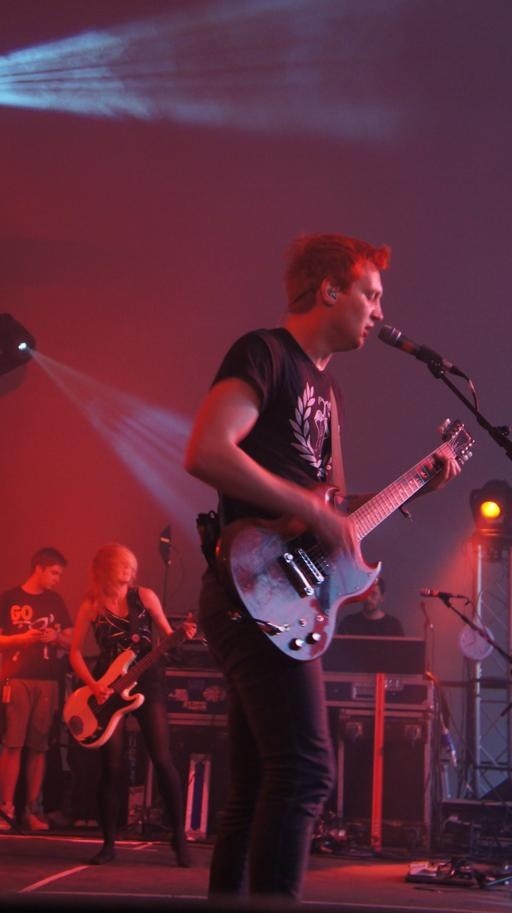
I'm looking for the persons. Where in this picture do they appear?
[0,546,76,832]
[184,226,463,912]
[70,543,197,870]
[338,577,406,672]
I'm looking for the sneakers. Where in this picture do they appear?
[0,804,14,830]
[20,812,48,832]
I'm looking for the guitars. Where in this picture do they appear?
[63,608,203,750]
[215,418,476,661]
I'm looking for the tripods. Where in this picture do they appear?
[122,578,181,843]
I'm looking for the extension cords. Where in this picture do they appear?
[406,871,475,886]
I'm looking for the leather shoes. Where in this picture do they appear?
[170,833,191,866]
[89,845,116,863]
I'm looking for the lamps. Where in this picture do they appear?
[0,315,36,375]
[469,480,512,538]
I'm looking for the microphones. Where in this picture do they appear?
[420,588,464,599]
[378,324,465,378]
[420,601,433,629]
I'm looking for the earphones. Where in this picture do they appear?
[328,288,336,299]
